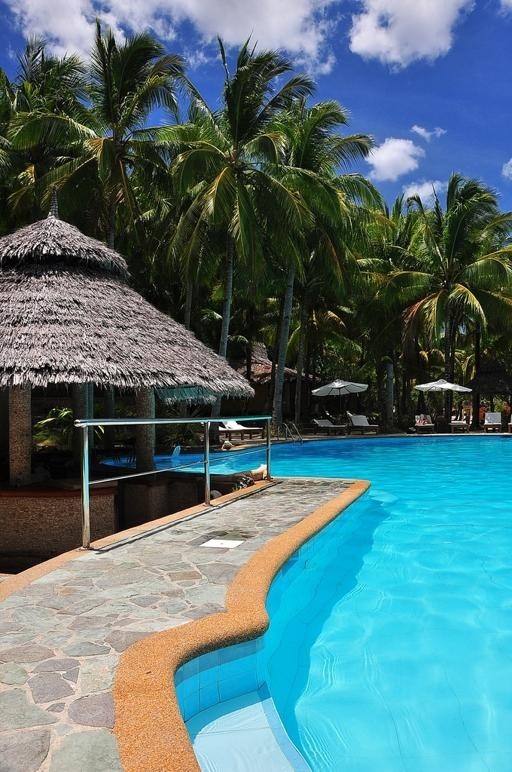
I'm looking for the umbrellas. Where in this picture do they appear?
[413,379,472,417]
[311,378,368,424]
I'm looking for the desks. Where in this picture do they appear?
[415,423,435,434]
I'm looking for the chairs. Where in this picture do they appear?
[313,418,347,436]
[218,426,245,441]
[484,412,502,433]
[222,421,265,439]
[415,414,433,434]
[507,414,512,434]
[114,473,201,533]
[347,414,379,435]
[171,446,181,457]
